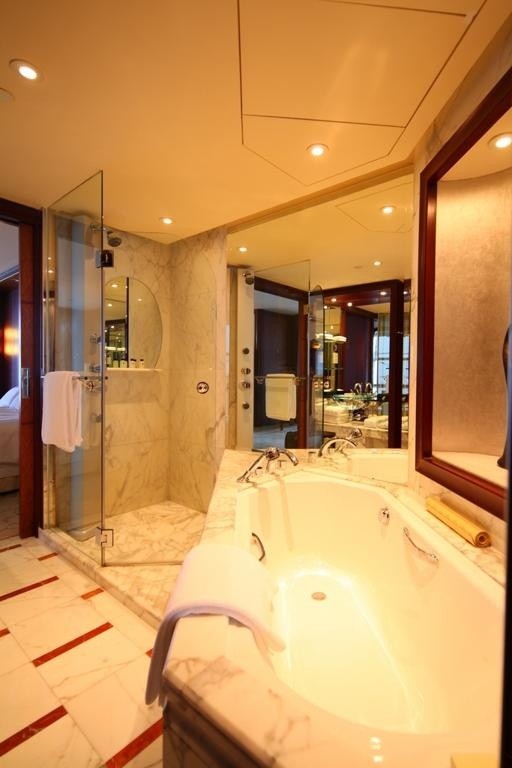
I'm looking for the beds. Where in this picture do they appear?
[0,385,20,492]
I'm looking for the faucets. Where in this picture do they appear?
[317,427,362,464]
[236,445,299,484]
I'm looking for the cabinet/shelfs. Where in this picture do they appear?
[158,683,257,767]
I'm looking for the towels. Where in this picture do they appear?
[40,369,84,452]
[264,371,298,421]
[144,538,290,706]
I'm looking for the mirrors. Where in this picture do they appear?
[323,295,410,404]
[101,274,164,369]
[224,174,419,452]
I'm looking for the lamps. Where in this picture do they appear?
[317,300,347,346]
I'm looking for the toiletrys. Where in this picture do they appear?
[106,350,145,368]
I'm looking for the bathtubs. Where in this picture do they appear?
[158,449,505,768]
